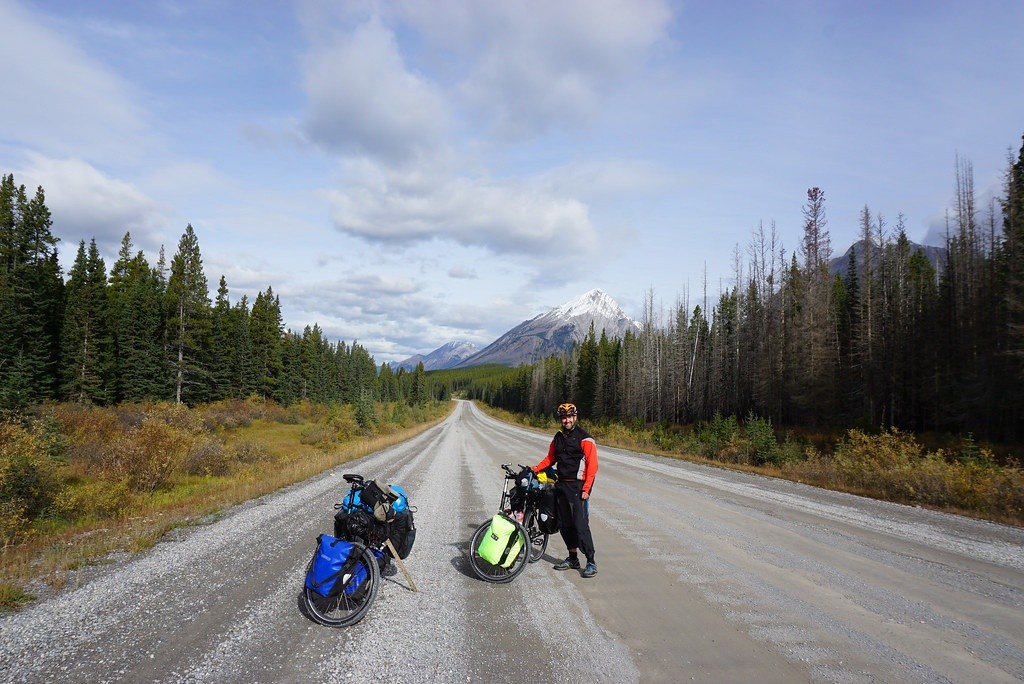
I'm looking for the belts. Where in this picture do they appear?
[559,479,576,481]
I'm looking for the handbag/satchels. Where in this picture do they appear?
[510,466,559,512]
[305,534,386,604]
[334,485,418,559]
[478,515,524,570]
[538,484,560,534]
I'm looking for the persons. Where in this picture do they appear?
[529,403,598,577]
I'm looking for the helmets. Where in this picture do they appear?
[557,403,577,417]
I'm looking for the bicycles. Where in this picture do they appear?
[467,463,550,583]
[299,473,400,629]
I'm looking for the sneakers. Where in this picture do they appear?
[554,557,580,570]
[582,562,598,577]
